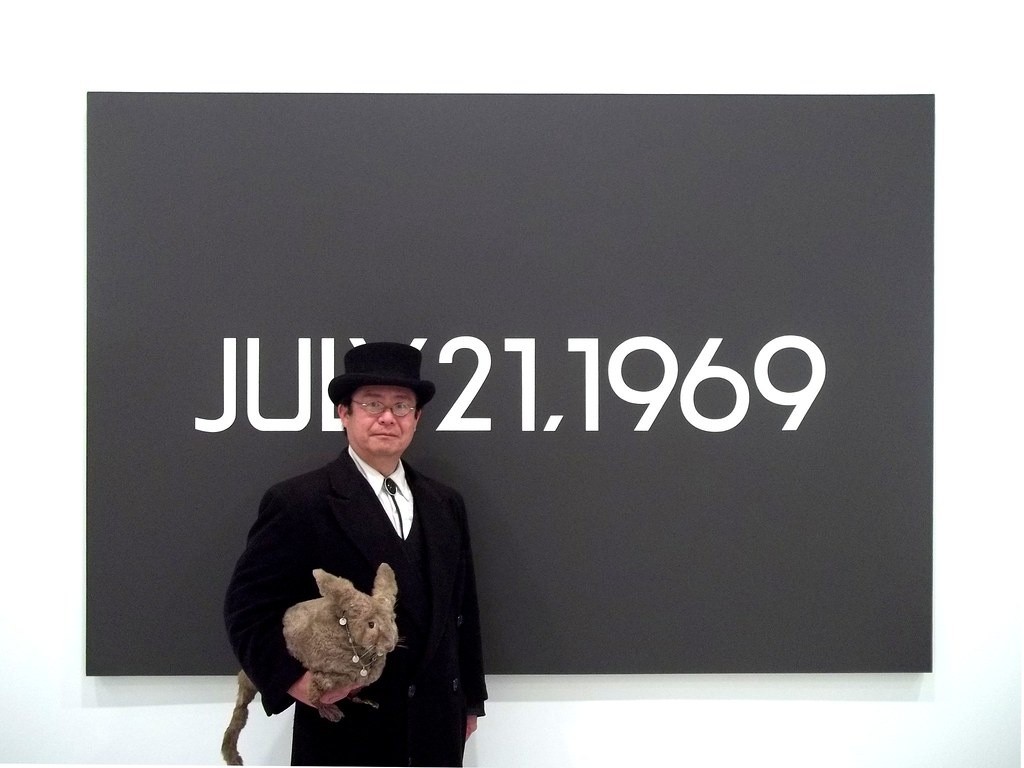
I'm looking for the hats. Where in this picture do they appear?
[328,342,435,411]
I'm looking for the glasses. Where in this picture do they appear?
[352,400,416,417]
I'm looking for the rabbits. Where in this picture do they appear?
[218,562,410,766]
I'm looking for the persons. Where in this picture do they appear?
[224,341,487,766]
